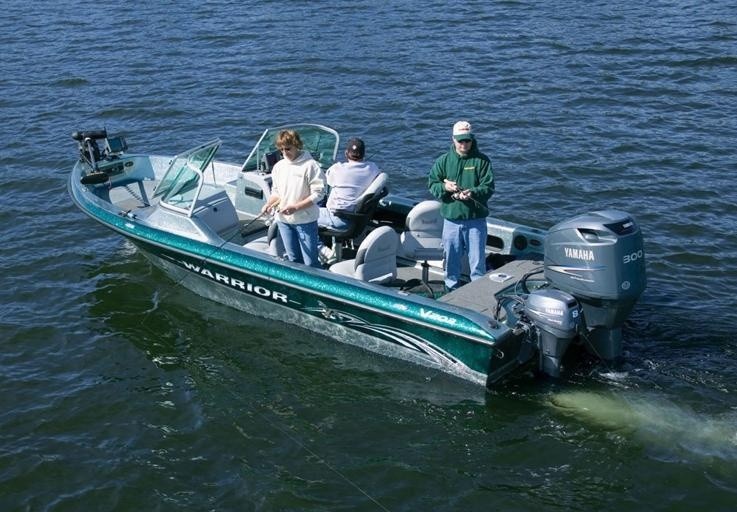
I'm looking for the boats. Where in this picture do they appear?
[62,126,654,402]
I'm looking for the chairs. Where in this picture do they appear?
[330,225,399,286]
[399,200,446,297]
[317,169,388,262]
[244,231,323,263]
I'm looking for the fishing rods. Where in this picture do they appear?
[443,179,504,223]
[363,127,410,162]
[141,199,280,324]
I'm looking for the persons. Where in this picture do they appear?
[316,138,381,266]
[261,129,328,268]
[428,121,495,292]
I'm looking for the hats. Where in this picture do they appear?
[346,138,365,156]
[452,121,473,141]
